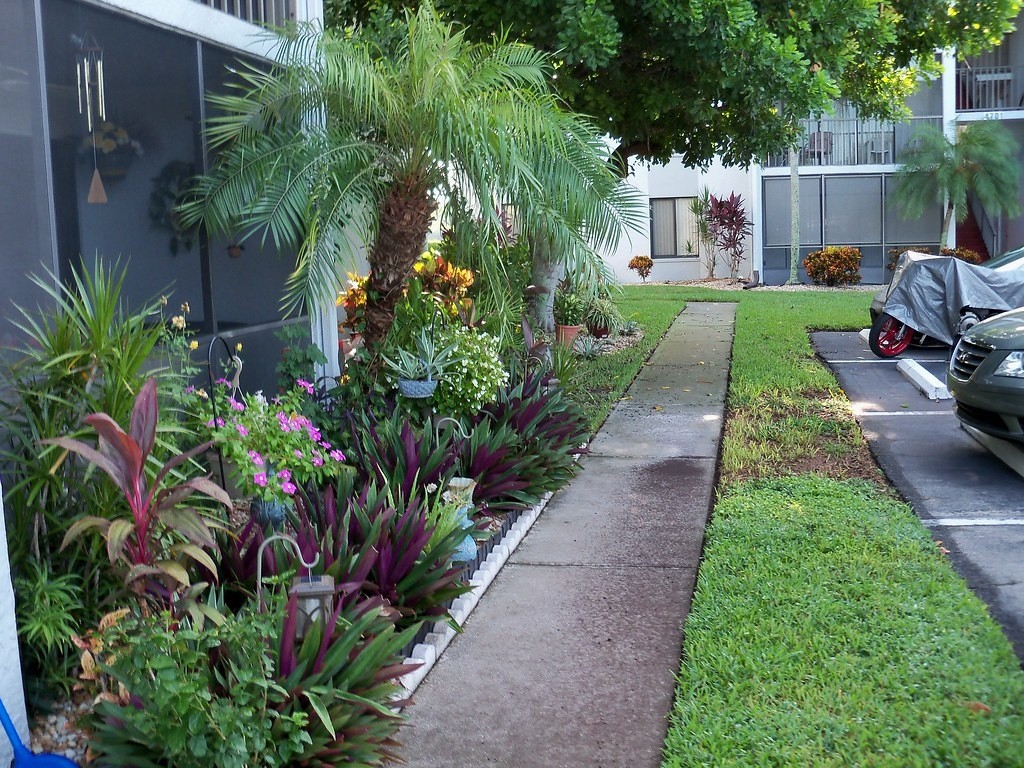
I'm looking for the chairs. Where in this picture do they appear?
[909,139,927,157]
[866,138,890,164]
[801,132,833,165]
[775,148,801,166]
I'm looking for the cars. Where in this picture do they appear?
[945,306,1024,445]
[869,243,1024,348]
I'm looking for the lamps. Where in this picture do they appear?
[289,576,334,641]
[543,378,562,396]
[447,476,478,561]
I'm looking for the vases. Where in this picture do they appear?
[206,451,267,499]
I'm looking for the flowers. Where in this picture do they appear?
[160,296,347,499]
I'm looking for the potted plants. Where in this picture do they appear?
[554,292,586,349]
[380,325,473,399]
[585,299,623,339]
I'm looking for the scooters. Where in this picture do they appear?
[868,250,957,359]
